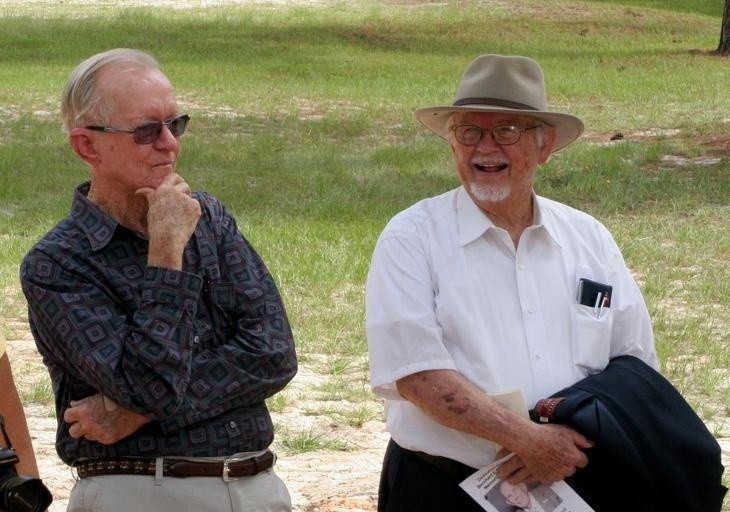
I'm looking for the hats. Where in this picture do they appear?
[414,54,583,153]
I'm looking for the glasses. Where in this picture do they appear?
[84,114,190,144]
[448,123,542,146]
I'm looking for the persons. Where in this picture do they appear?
[365,53,660,511]
[20,54,298,512]
[499,478,560,510]
[0,329,52,512]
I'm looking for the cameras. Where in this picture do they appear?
[0,447,53,512]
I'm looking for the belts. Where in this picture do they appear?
[76,450,278,482]
